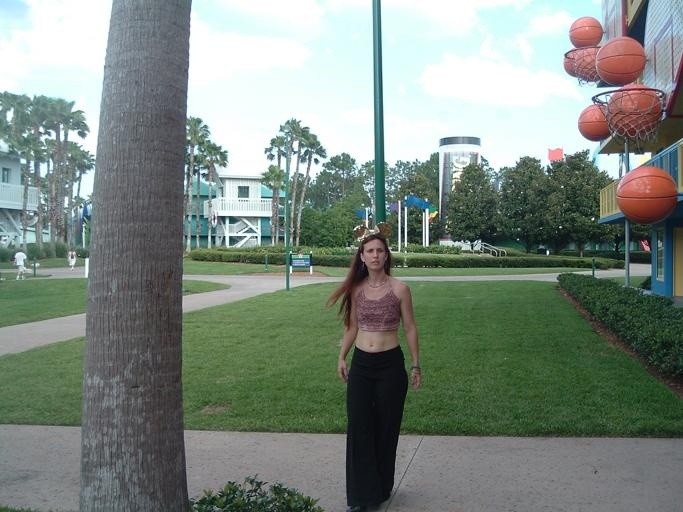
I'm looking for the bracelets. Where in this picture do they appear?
[410,367,420,375]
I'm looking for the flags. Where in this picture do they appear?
[82,203,89,228]
[428,204,437,222]
[355,209,365,219]
[548,148,563,161]
[405,195,429,208]
[389,203,398,213]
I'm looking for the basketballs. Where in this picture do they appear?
[615,167,678,224]
[564,18,661,141]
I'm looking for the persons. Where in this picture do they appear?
[323,232,421,512]
[13,248,27,280]
[66,246,77,271]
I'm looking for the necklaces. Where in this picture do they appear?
[365,275,387,289]
